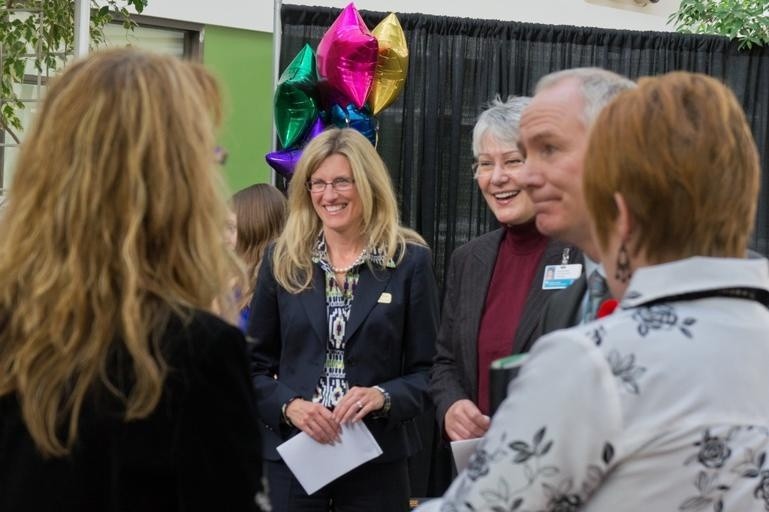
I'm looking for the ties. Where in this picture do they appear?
[584,271,608,320]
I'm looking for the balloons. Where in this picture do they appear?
[265,1,410,182]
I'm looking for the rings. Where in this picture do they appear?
[356,400,363,409]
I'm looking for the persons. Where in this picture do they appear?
[241,126,439,512]
[214,184,289,333]
[432,94,583,442]
[0,45,264,510]
[411,69,767,512]
[515,68,636,346]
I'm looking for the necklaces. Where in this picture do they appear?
[332,251,367,273]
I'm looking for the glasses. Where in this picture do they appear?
[471,158,527,176]
[306,176,354,193]
